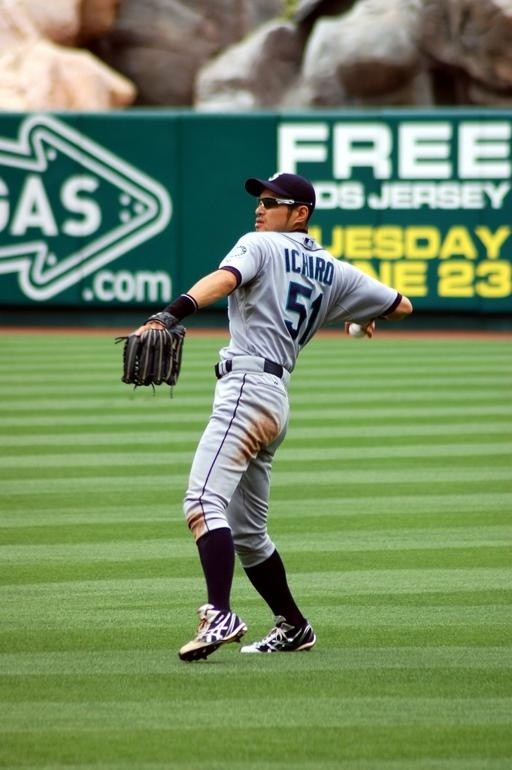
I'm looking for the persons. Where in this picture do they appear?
[124,171,416,661]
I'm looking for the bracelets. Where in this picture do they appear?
[163,291,199,327]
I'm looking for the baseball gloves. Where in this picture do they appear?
[114,312,185,398]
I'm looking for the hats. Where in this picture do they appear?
[244,171,317,211]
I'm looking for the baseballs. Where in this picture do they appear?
[349,323,366,337]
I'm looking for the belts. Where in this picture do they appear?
[213,355,292,387]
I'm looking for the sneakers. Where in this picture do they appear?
[178,603,248,662]
[240,615,317,654]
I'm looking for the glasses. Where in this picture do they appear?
[258,197,313,210]
